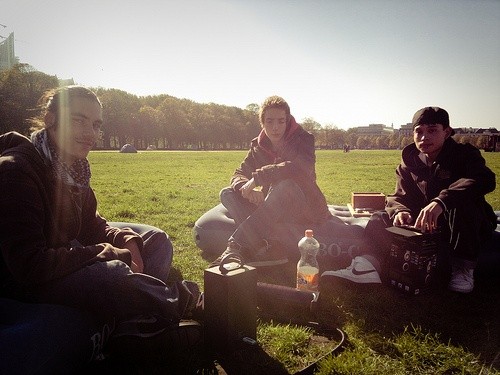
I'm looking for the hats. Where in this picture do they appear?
[413,108,456,135]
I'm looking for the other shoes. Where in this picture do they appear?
[106,316,204,361]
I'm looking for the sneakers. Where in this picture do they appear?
[319,255,381,291]
[449,264,476,292]
[212,234,250,265]
[244,237,288,267]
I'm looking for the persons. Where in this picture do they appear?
[0,86,202,337]
[319,107,498,294]
[208,98,328,269]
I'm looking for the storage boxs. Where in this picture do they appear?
[351,192,386,212]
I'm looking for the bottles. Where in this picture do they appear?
[296,230,320,302]
